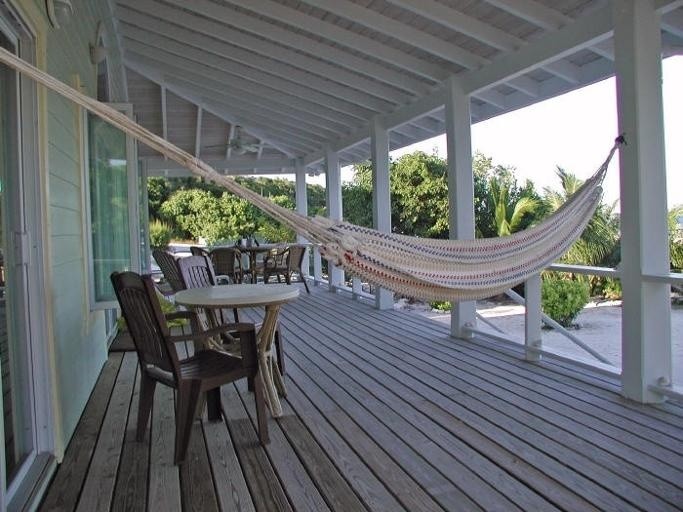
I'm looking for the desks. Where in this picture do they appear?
[175,282,300,419]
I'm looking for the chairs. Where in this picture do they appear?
[190,236,312,294]
[104,249,286,466]
